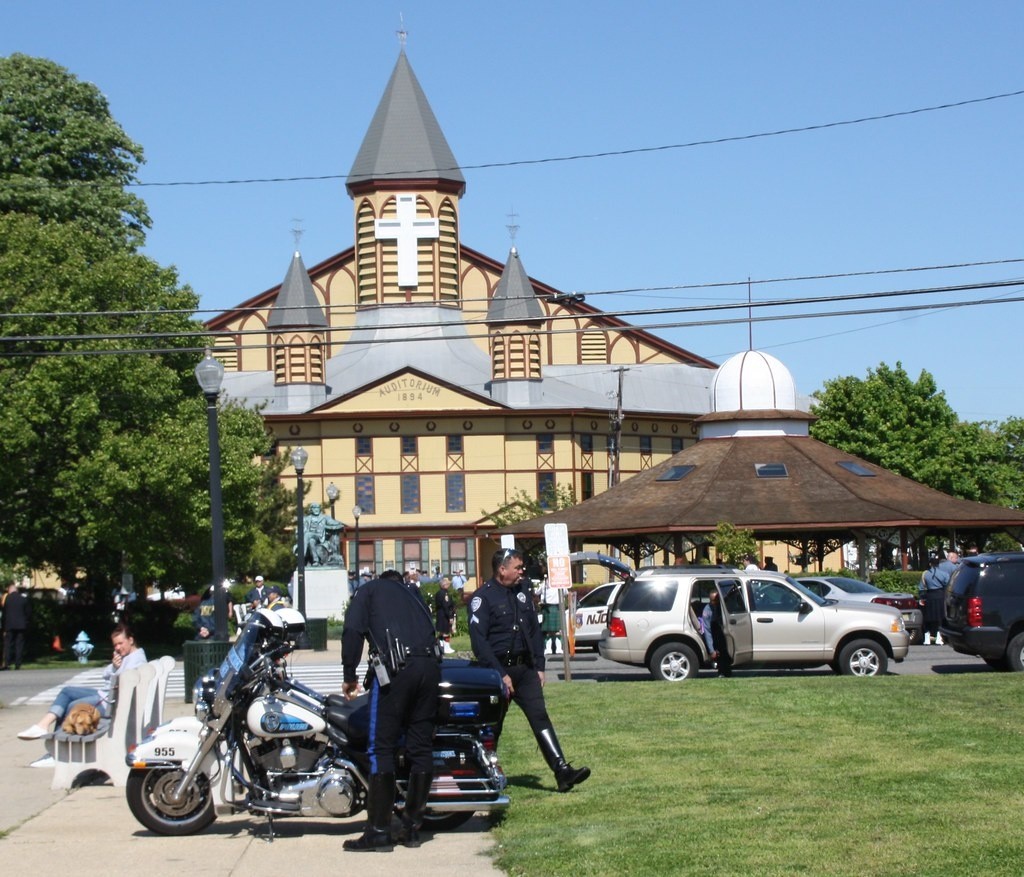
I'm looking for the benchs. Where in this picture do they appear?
[234,601,253,636]
[38,653,176,790]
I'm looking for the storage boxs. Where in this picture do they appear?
[439,659,505,725]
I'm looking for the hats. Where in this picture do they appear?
[265,585,280,597]
[255,575,263,581]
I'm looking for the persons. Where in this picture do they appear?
[17,626,148,768]
[466,548,591,793]
[347,567,442,593]
[245,576,269,608]
[702,590,732,677]
[763,556,777,571]
[743,555,760,570]
[919,555,949,645]
[193,587,233,641]
[342,569,439,851]
[940,546,978,574]
[114,599,125,623]
[533,578,568,653]
[435,578,456,654]
[0,586,32,671]
[267,585,284,611]
[453,570,466,597]
[303,504,345,566]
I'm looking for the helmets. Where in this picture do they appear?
[257,609,284,637]
[276,607,306,632]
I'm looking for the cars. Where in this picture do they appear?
[939,552,1024,672]
[566,550,923,681]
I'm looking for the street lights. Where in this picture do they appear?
[195,344,230,643]
[327,481,338,520]
[352,504,361,582]
[291,445,308,618]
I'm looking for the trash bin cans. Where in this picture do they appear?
[183,641,235,704]
[539,603,560,632]
[306,619,328,650]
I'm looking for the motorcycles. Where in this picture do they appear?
[125,608,511,841]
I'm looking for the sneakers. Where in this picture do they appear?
[17,725,49,740]
[30,753,55,767]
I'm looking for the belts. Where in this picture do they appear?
[383,646,435,662]
[499,654,533,666]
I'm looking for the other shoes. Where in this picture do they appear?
[718,669,727,678]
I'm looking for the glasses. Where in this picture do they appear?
[502,548,513,564]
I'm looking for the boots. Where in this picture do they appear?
[533,726,591,791]
[936,631,944,644]
[442,640,455,653]
[342,773,394,852]
[440,640,444,647]
[923,631,930,645]
[394,771,434,847]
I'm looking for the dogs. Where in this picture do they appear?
[61,702,101,735]
